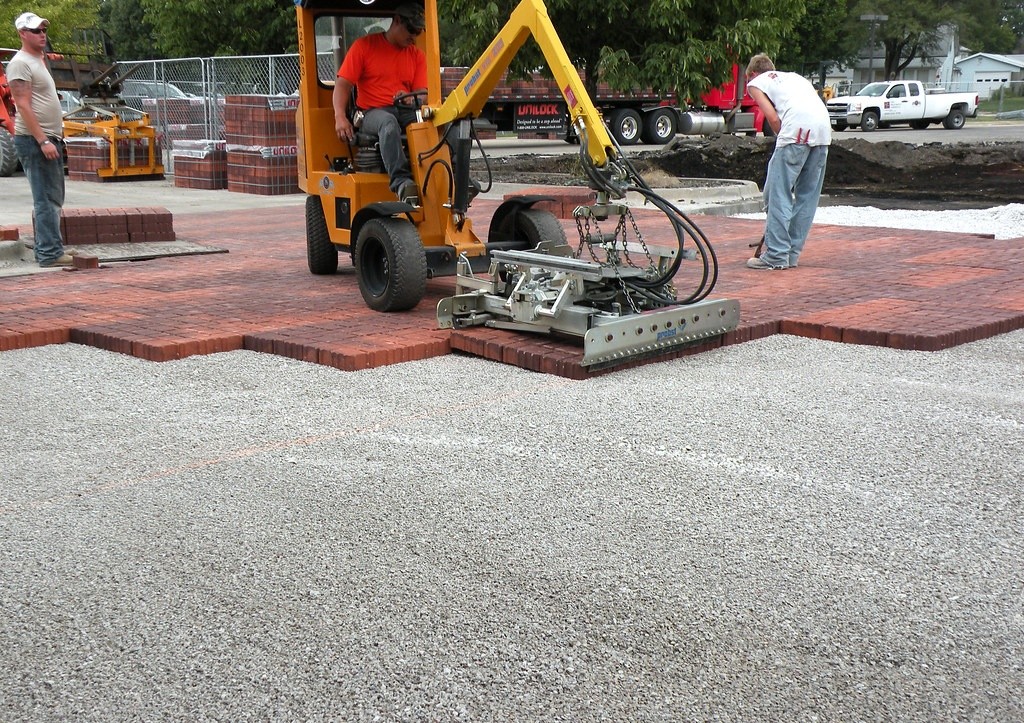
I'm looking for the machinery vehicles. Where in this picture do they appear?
[0,27,164,179]
[295,1,741,366]
[813,82,836,104]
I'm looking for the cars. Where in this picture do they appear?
[123,82,202,108]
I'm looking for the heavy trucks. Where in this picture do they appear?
[440,42,778,145]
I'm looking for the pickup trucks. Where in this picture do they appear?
[826,80,979,132]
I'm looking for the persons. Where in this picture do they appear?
[5,11,77,266]
[332,3,482,207]
[745,54,832,268]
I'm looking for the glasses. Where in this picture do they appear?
[23,28,47,34]
[399,14,421,36]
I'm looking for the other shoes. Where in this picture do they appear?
[747,257,786,270]
[40,253,73,266]
[64,249,78,255]
[397,180,415,202]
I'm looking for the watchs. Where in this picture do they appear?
[39,138,51,146]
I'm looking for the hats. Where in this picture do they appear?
[14,13,50,29]
[398,2,425,29]
